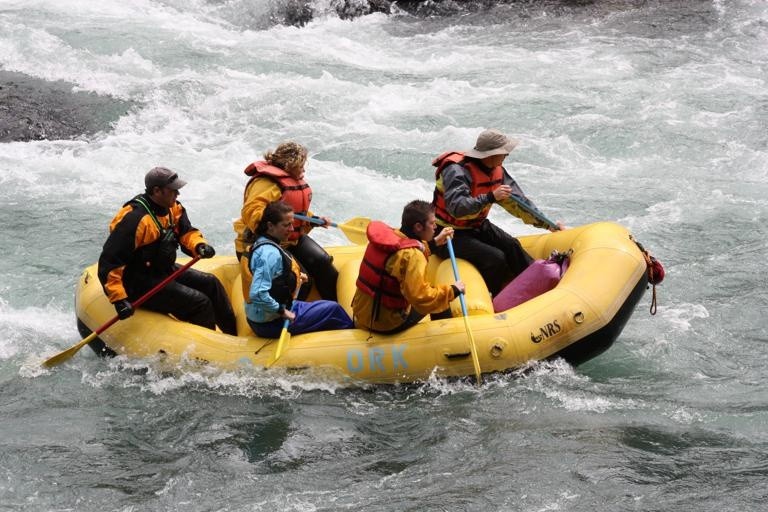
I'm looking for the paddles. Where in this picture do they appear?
[445,234,482,387]
[293,214,371,246]
[261,276,302,368]
[38,255,201,368]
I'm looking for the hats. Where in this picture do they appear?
[145,166,186,192]
[464,130,518,160]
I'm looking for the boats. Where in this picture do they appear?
[74,217,669,382]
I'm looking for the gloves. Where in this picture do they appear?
[197,243,214,258]
[115,300,134,320]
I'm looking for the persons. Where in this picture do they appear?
[98,166,237,336]
[349,199,465,335]
[240,200,355,338]
[233,140,337,302]
[426,130,565,299]
